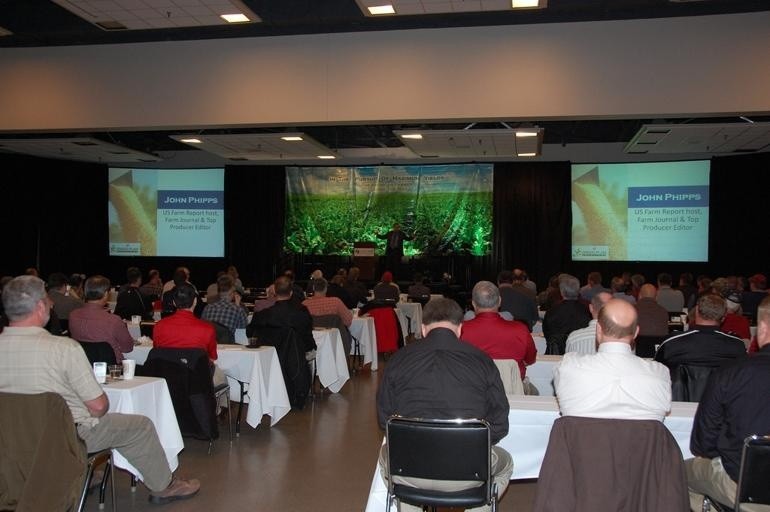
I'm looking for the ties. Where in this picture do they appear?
[391,231,397,249]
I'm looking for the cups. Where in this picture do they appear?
[352,308,360,317]
[680,315,688,325]
[121,360,136,378]
[132,315,141,324]
[249,337,257,344]
[93,362,107,383]
[108,365,123,380]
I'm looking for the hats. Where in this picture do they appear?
[750,273,767,289]
[383,271,393,282]
[497,270,513,281]
[710,278,729,292]
[724,289,745,303]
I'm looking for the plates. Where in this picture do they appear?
[245,345,261,348]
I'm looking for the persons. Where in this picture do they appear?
[697,276,745,292]
[552,298,673,420]
[583,271,611,300]
[656,273,684,312]
[497,270,538,332]
[542,275,593,337]
[142,268,163,295]
[523,270,537,293]
[26,267,39,278]
[654,293,747,361]
[247,275,317,351]
[114,267,151,319]
[284,269,305,301]
[460,280,539,395]
[46,273,83,320]
[71,273,85,300]
[408,272,430,297]
[328,274,353,309]
[375,297,514,512]
[0,275,201,505]
[565,291,637,354]
[632,283,670,336]
[508,267,536,305]
[348,267,368,297]
[743,274,767,310]
[201,274,248,343]
[375,223,416,256]
[611,271,645,303]
[206,271,227,304]
[163,267,200,295]
[686,295,751,339]
[70,274,134,364]
[374,271,398,299]
[683,297,770,512]
[163,271,201,316]
[152,285,218,361]
[337,268,348,274]
[228,266,243,288]
[301,277,353,327]
[306,269,323,293]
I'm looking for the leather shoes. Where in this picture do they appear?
[88,470,105,495]
[148,476,201,505]
